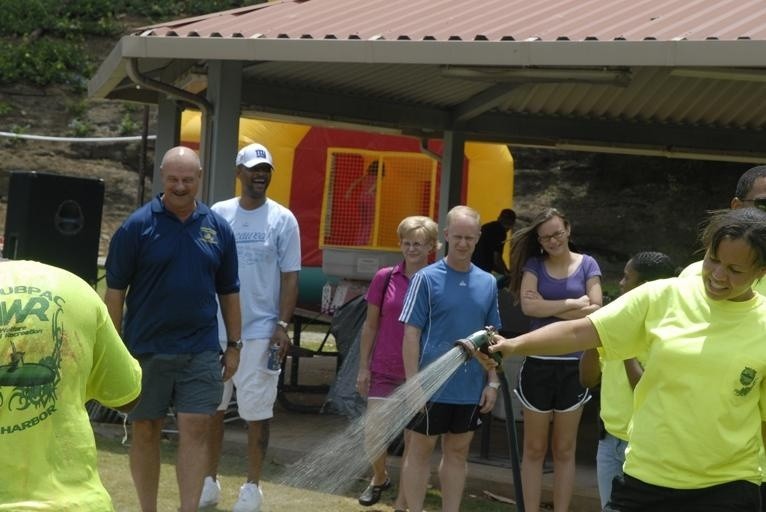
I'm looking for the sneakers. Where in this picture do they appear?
[198,475,223,509]
[231,482,264,511]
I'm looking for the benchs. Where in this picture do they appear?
[277,344,318,412]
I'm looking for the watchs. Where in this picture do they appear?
[487,382,502,392]
[226,340,243,350]
[277,320,289,332]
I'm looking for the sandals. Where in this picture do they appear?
[358,475,392,506]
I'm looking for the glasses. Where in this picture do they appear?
[536,227,565,243]
[739,196,766,211]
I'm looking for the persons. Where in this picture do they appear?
[579,252,684,509]
[0,258,144,512]
[346,161,385,247]
[198,144,301,512]
[105,145,242,512]
[355,215,442,505]
[397,204,503,512]
[677,165,765,299]
[503,207,604,512]
[471,209,517,291]
[474,207,765,512]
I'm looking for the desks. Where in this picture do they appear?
[292,308,335,386]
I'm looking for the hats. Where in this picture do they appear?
[236,142,275,173]
